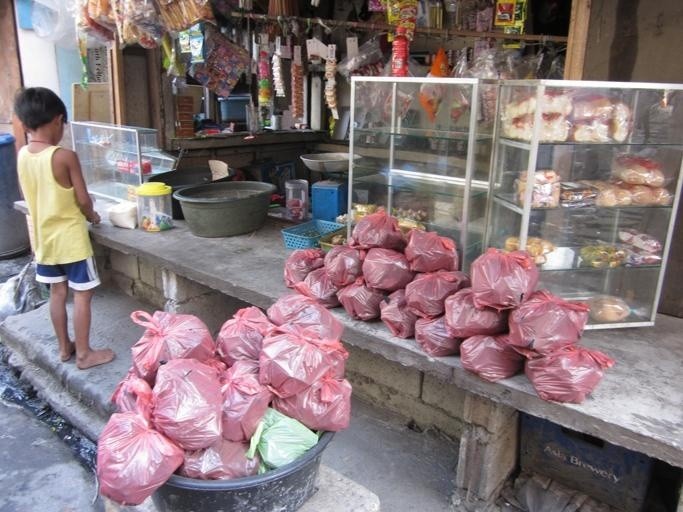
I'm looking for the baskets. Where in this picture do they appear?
[281,218,345,249]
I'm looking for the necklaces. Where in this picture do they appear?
[30,139,51,145]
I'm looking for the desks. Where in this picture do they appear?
[10,195,683,512]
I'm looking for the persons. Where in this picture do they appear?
[12,84,116,369]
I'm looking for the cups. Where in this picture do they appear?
[284,179,309,222]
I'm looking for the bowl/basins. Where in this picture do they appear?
[170,180,278,239]
[298,152,361,173]
[146,427,336,512]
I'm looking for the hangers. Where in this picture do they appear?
[222,10,567,68]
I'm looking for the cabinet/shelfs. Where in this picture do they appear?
[484,77,681,329]
[67,119,172,208]
[350,77,499,271]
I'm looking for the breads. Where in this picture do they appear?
[505,237,554,265]
[502,92,674,209]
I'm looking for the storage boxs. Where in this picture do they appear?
[217,97,249,121]
